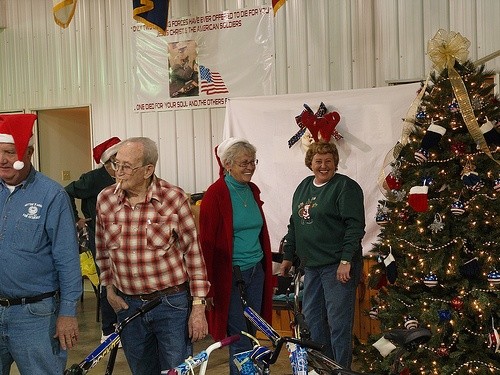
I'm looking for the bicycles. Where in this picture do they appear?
[232,264,325,375]
[62,295,163,375]
[286,300,431,375]
[160,333,242,375]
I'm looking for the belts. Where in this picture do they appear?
[0,287,60,308]
[128,280,190,302]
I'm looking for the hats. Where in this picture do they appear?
[0,114,38,170]
[214,137,249,178]
[93,137,122,164]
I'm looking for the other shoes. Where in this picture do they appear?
[100,331,122,347]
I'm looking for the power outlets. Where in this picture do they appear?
[62,170,70,181]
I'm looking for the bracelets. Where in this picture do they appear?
[341,260,350,264]
[193,300,207,306]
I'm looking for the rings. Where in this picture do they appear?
[203,334,206,335]
[71,335,75,339]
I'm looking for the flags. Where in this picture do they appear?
[53,0,77,29]
[272,0,286,17]
[133,0,170,36]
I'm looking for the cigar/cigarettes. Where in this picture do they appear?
[114,179,122,194]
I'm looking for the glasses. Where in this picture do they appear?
[233,159,258,167]
[110,162,152,173]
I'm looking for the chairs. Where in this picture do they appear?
[79,223,101,323]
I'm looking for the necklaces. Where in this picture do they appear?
[225,176,249,207]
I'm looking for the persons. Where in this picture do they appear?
[65,137,123,348]
[280,142,366,375]
[95,137,210,375]
[198,140,273,375]
[0,114,82,375]
